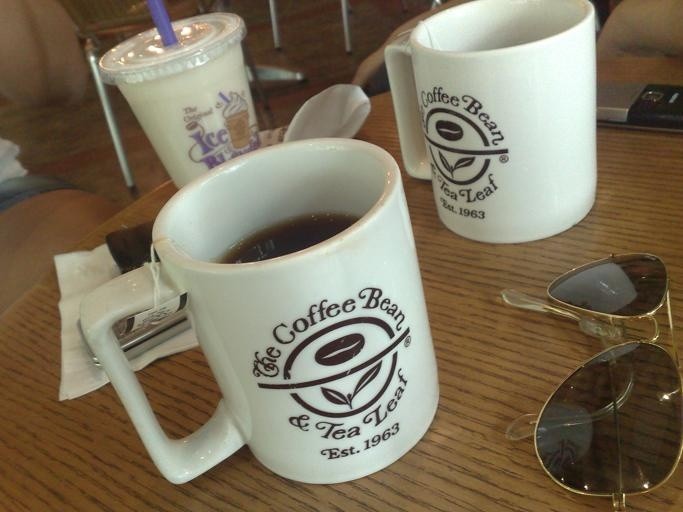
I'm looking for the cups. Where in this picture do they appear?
[95,12,265,199]
[74,133,442,487]
[382,0,598,246]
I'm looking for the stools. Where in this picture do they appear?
[0,189,118,314]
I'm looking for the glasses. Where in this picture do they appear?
[491,251,683,512]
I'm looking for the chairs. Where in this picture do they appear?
[53,0,273,195]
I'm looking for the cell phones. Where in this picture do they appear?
[76,292,191,369]
[597,82,683,129]
